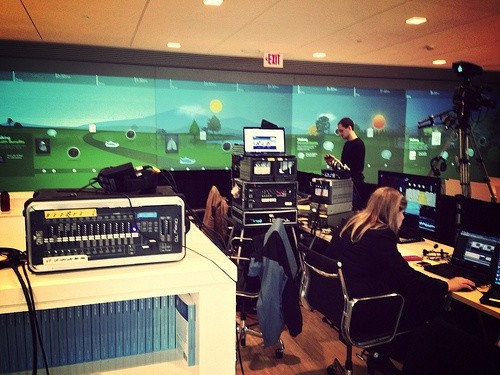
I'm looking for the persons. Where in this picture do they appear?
[324,118,365,210]
[327,186,475,375]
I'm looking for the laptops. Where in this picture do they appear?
[243,126,297,159]
[480,252,500,309]
[424,225,500,287]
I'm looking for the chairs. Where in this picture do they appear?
[296,242,435,375]
[229,218,285,359]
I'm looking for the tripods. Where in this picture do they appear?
[417,84,499,203]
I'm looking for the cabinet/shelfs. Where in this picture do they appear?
[0,216,240,375]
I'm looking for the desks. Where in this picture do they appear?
[296,213,500,320]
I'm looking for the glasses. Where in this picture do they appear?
[338,128,345,133]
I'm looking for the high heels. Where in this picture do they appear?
[366,356,383,375]
[383,360,411,375]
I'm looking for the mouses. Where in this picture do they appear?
[454,284,476,292]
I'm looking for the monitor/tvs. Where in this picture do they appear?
[377,169,441,244]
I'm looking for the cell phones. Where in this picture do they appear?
[415,261,430,269]
